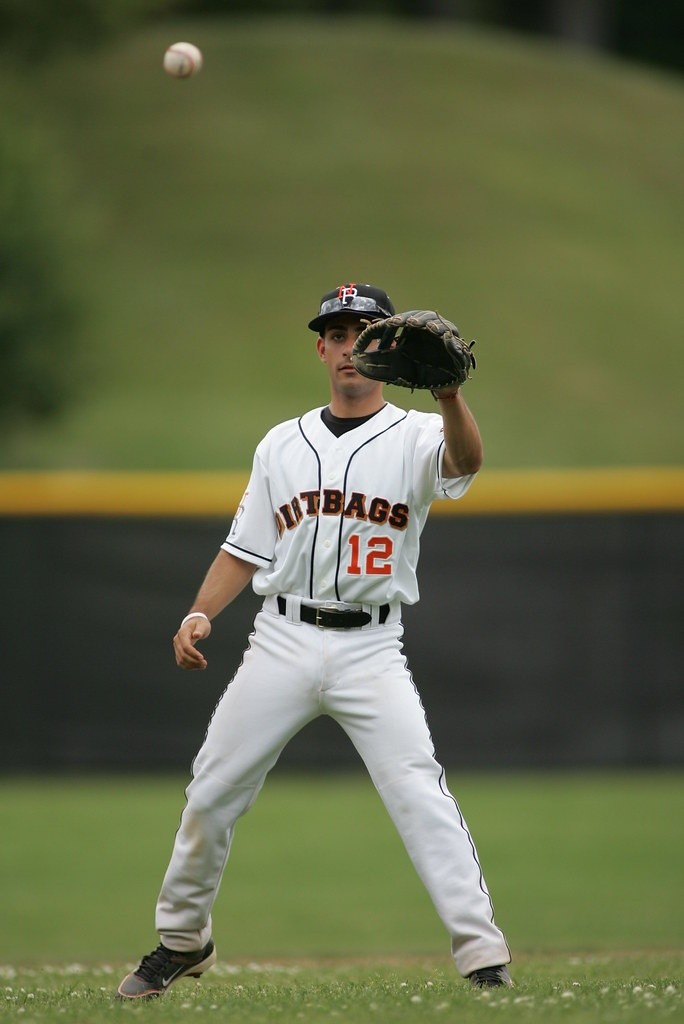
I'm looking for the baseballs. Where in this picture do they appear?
[162,42,204,80]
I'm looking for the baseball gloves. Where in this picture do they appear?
[353,308,477,396]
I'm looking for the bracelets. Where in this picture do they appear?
[181,611,209,628]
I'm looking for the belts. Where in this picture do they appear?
[277,595,390,628]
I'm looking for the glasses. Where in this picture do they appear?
[317,296,392,316]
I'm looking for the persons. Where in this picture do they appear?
[116,285,516,1002]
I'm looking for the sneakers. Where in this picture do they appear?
[469,965,511,989]
[116,937,216,1002]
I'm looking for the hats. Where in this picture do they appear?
[308,284,395,332]
